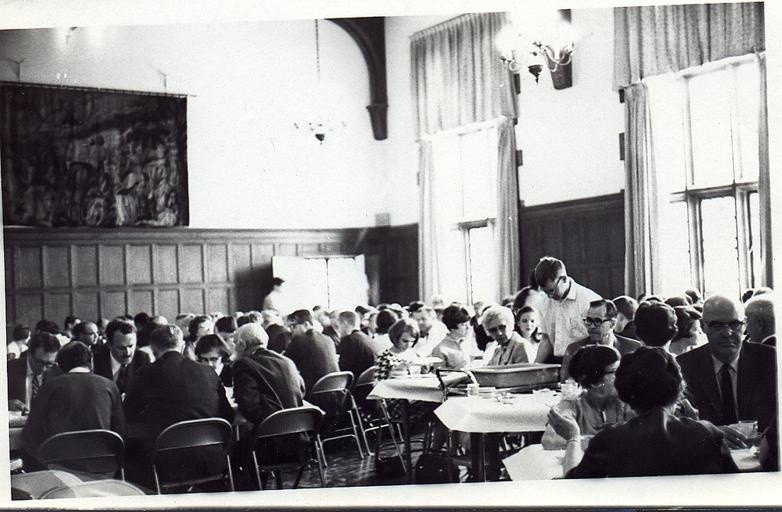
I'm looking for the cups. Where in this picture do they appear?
[466,383,480,396]
[739,420,758,440]
[409,365,421,379]
[560,384,574,391]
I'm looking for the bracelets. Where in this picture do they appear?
[566,439,581,446]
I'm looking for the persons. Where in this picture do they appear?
[534,257,604,363]
[542,344,636,449]
[615,288,779,471]
[263,277,286,312]
[547,346,738,479]
[559,299,641,387]
[7,306,382,481]
[372,269,540,466]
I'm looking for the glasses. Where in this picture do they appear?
[582,316,616,327]
[599,367,619,376]
[488,324,507,333]
[543,275,566,295]
[287,322,300,330]
[199,352,223,363]
[30,354,57,369]
[701,318,745,332]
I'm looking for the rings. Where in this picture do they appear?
[726,433,732,438]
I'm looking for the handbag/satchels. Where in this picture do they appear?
[413,448,461,484]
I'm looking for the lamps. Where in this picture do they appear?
[491,9,583,92]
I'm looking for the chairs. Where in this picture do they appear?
[10,300,782,498]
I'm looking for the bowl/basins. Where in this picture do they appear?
[478,386,496,399]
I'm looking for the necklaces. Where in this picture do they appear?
[588,393,609,423]
